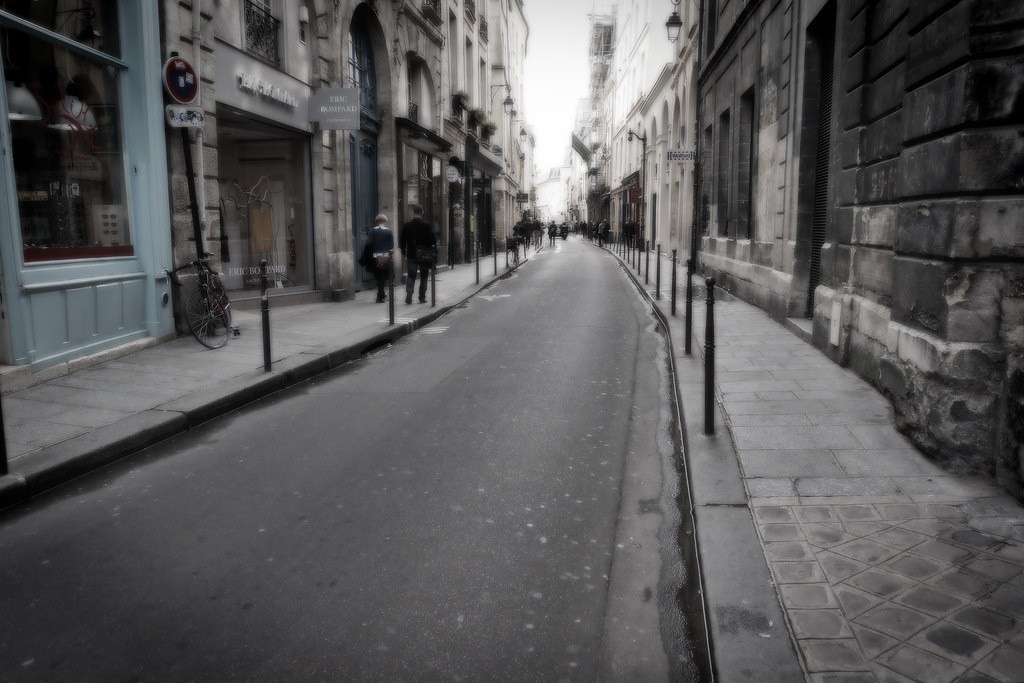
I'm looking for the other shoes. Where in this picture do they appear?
[376,295,387,303]
[405,301,411,304]
[420,300,427,303]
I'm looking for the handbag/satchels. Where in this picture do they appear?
[416,248,437,264]
[377,256,394,270]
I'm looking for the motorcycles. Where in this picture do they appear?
[559,226,568,240]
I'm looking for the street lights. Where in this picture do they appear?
[627,127,647,239]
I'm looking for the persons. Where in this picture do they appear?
[510,211,635,250]
[362,213,396,303]
[400,204,438,304]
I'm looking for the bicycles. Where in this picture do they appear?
[506,235,520,272]
[165,251,240,349]
